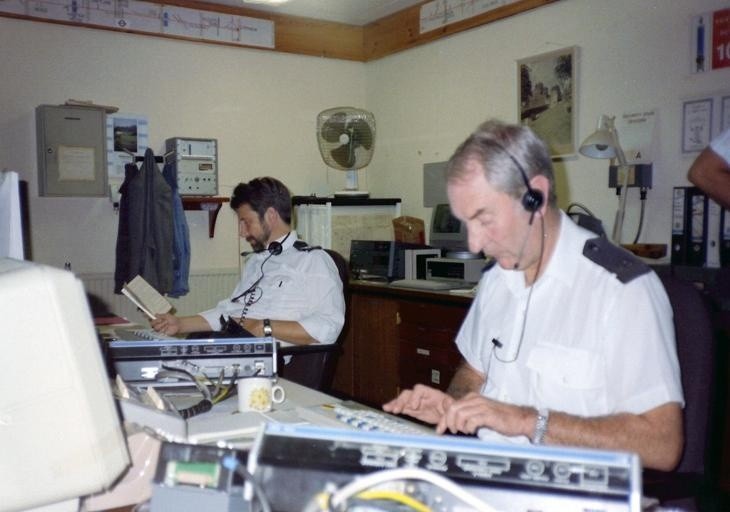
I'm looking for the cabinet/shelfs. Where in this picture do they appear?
[319,283,476,436]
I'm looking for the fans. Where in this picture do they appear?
[317,107,376,199]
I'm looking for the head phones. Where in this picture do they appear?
[268,232,290,255]
[486,138,543,212]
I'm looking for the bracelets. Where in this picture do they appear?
[531,405,551,444]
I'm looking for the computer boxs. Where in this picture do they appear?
[426,257,488,283]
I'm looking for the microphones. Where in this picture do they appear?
[241,247,276,256]
[514,200,540,270]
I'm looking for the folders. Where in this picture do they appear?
[672,187,730,270]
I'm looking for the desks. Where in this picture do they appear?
[80,372,688,511]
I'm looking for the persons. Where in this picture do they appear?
[140,174,347,387]
[685,124,729,214]
[381,118,689,473]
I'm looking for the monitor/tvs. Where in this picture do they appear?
[429,202,476,259]
[0,255,131,511]
[0,170,25,260]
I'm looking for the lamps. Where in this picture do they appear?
[578,113,631,247]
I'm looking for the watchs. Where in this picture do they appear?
[261,318,274,340]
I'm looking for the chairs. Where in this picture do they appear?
[278,248,347,391]
[643,264,716,512]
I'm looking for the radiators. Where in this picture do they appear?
[75,269,240,341]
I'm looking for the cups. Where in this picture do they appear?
[237,376,286,414]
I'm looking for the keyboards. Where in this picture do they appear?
[294,400,442,436]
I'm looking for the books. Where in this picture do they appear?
[120,275,173,322]
